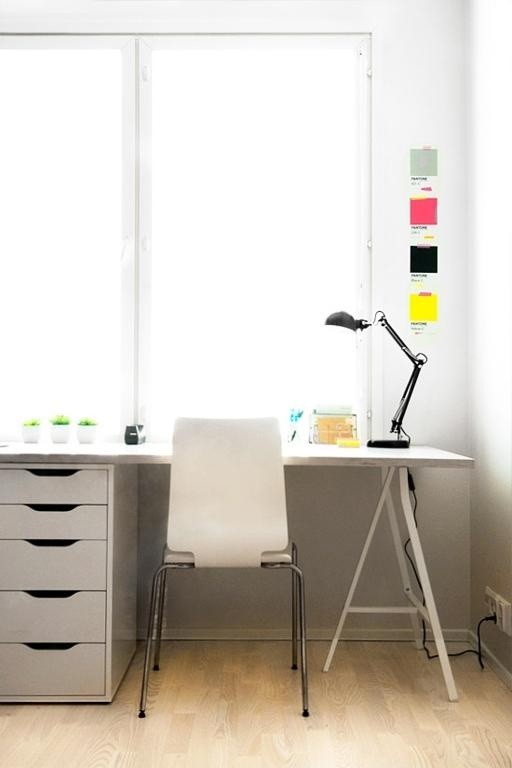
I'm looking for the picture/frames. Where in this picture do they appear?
[301,403,369,452]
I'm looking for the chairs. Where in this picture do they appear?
[136,414,311,720]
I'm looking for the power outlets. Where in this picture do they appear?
[482,584,512,637]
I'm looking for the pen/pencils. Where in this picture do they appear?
[288,409,303,441]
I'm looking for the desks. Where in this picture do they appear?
[1,441,478,705]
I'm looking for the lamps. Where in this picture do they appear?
[319,304,430,452]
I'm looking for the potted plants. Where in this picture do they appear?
[18,414,105,448]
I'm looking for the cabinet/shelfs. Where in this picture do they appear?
[0,462,140,706]
